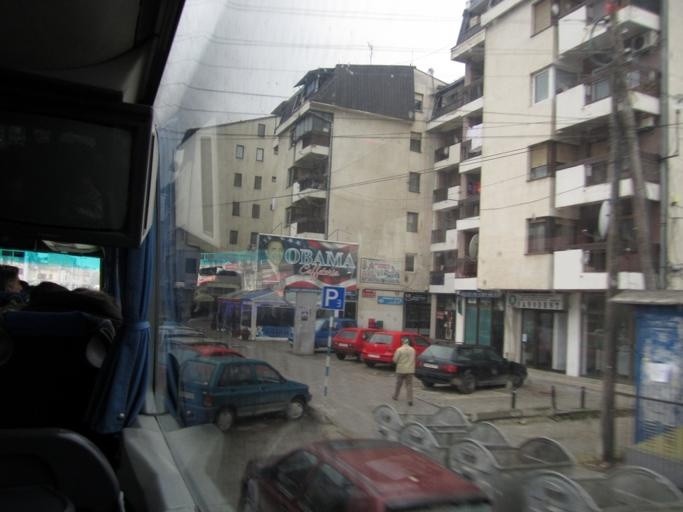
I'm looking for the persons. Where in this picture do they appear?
[390,338,415,406]
[259,239,288,282]
[0,265,26,296]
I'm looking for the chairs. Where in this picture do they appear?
[0,429,124,512]
[0,311,130,453]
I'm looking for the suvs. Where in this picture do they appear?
[287,319,358,352]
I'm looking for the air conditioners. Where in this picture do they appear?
[631,30,658,57]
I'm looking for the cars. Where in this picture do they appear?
[414,342,529,394]
[240,438,490,512]
[175,356,314,431]
[333,327,394,363]
[361,330,436,369]
[155,323,244,388]
